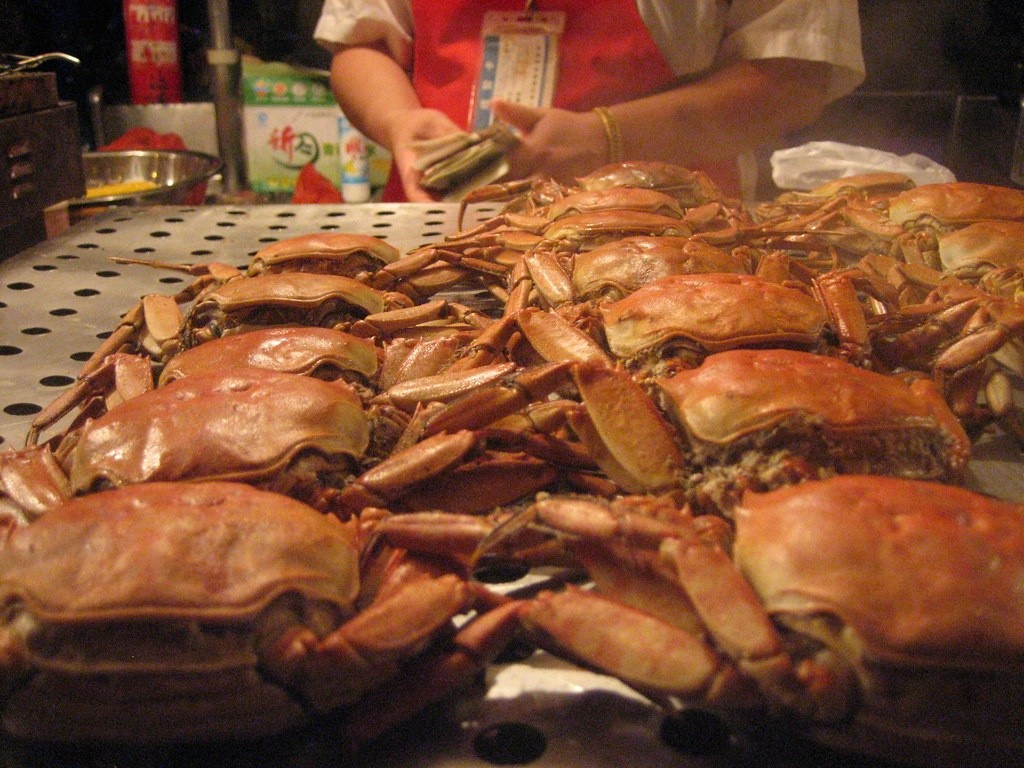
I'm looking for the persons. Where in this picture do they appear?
[313,0,867,203]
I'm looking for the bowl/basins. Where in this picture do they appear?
[67,149,225,226]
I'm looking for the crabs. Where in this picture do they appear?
[1,166,1022,747]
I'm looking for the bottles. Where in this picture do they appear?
[336,103,371,203]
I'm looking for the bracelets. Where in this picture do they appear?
[594,106,621,163]
